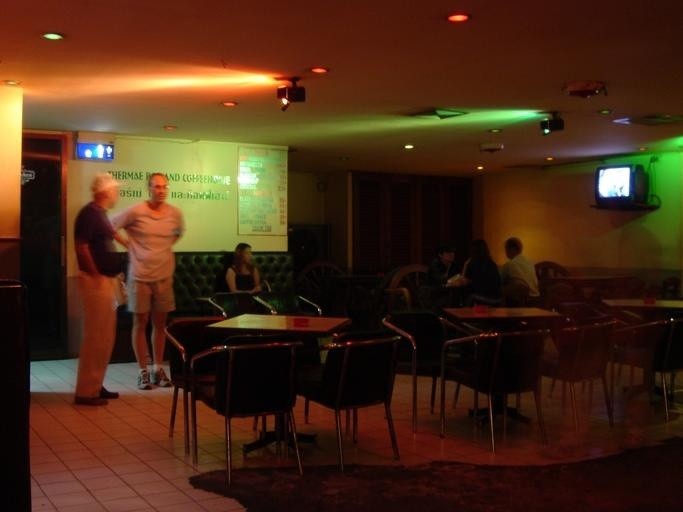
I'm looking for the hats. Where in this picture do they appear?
[91,173,118,196]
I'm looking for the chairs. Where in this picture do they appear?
[163,316,225,456]
[381,310,472,428]
[309,335,403,474]
[190,341,304,487]
[542,324,616,435]
[207,292,323,317]
[609,318,682,422]
[440,330,548,453]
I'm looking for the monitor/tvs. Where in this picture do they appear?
[594,164,647,205]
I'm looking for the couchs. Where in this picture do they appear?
[100,250,317,362]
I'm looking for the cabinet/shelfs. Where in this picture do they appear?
[326,168,481,276]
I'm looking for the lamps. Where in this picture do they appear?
[274,76,307,112]
[538,110,564,136]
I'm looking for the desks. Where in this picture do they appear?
[443,307,562,425]
[206,313,351,455]
[602,299,683,399]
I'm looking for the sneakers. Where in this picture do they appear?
[153,369,172,387]
[138,370,152,390]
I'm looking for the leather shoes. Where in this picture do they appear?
[75,397,107,406]
[100,386,118,398]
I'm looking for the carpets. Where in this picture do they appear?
[191,435,683,512]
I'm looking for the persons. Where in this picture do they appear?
[447,240,501,305]
[73,171,130,406]
[422,241,457,287]
[108,172,185,390]
[501,237,540,299]
[224,243,261,293]
